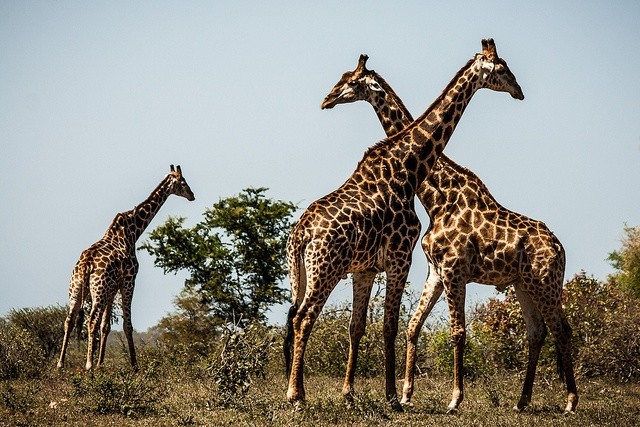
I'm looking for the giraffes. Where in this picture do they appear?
[57,164,195,375]
[321,53,579,415]
[283,38,524,411]
